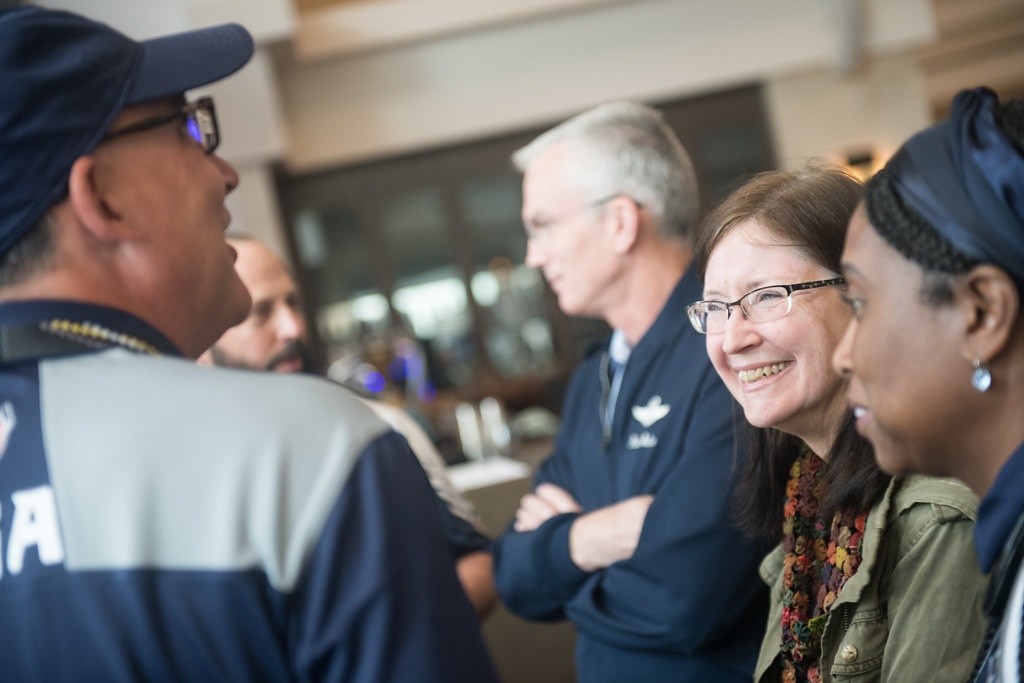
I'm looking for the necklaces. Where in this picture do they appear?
[46,320,160,357]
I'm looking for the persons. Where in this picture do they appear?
[693,162,990,683]
[828,87,1024,683]
[0,6,500,683]
[490,101,803,683]
[184,228,501,636]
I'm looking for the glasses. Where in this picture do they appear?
[101,97,220,154]
[684,277,846,335]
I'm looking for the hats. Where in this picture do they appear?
[878,85,1024,278]
[0,8,254,253]
